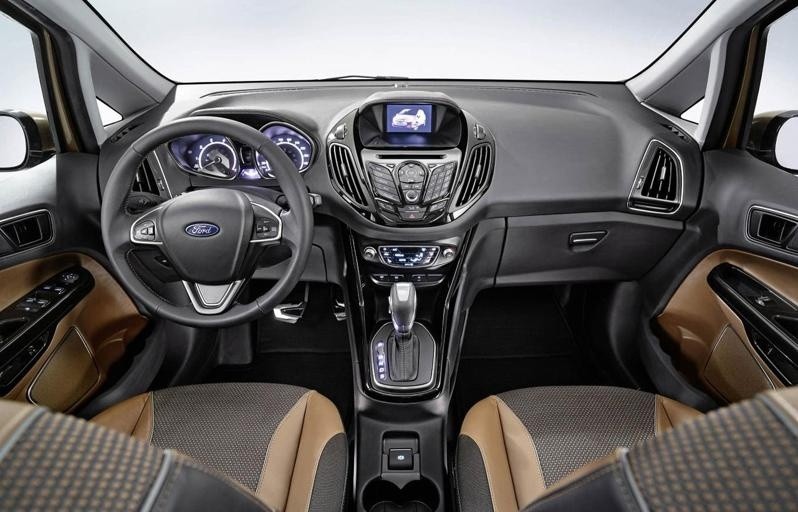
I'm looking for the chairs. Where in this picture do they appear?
[0,383,349,512]
[454,385,798,512]
